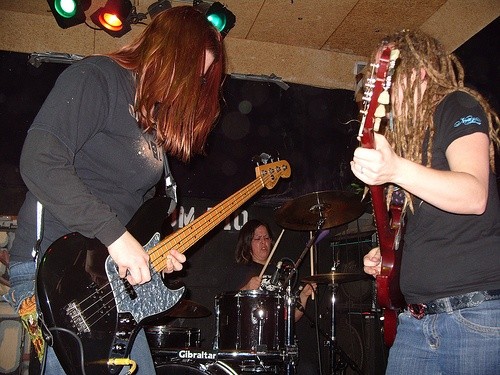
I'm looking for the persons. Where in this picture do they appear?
[217,218,317,322]
[4,5,227,375]
[349,29,500,375]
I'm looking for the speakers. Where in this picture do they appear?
[315,306,388,375]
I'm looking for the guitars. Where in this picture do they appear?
[355,45,407,343]
[35,152,293,374]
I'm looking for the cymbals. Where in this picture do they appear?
[280,188,365,229]
[167,299,213,320]
[299,271,369,284]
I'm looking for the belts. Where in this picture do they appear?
[408,289,500,320]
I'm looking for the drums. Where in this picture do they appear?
[217,291,298,359]
[153,355,239,375]
[142,326,202,350]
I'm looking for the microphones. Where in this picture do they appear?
[270,260,283,285]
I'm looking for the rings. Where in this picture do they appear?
[360,166,364,174]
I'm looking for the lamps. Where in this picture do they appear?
[148,0,172,22]
[89,0,137,40]
[192,0,237,41]
[46,0,92,31]
